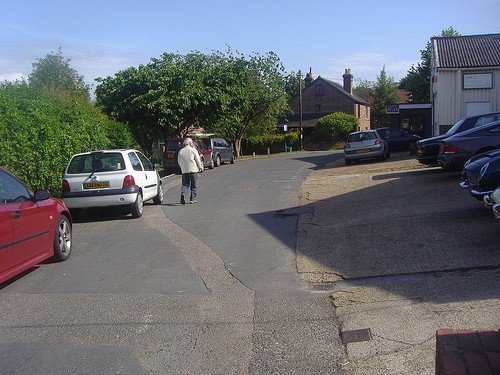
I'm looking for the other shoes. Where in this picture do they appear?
[181,194,186,205]
[190,199,197,204]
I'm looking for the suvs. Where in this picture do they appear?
[164,137,215,171]
[203,136,236,166]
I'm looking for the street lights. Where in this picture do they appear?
[296,69,304,151]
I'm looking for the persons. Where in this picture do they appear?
[178,138,204,205]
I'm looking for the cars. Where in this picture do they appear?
[342,131,388,164]
[484,188,499,218]
[375,127,420,150]
[0,165,75,290]
[64,147,164,216]
[437,121,500,167]
[460,148,500,200]
[414,113,500,166]
